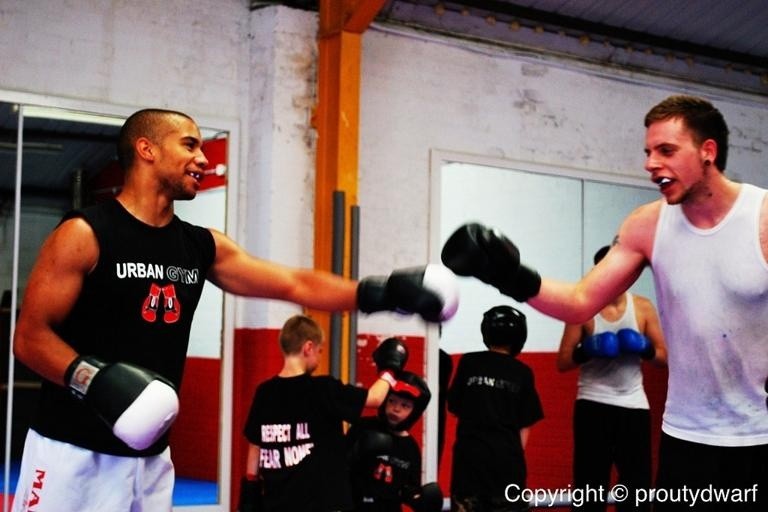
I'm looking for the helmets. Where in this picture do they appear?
[379,370,430,432]
[481,306,527,353]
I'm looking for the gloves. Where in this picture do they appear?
[573,329,655,365]
[373,338,408,389]
[357,263,458,321]
[441,224,541,303]
[64,352,181,450]
[412,482,443,511]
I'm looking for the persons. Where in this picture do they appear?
[344,372,442,512]
[555,245,668,512]
[441,94,768,512]
[439,324,453,464]
[12,109,458,512]
[240,316,408,512]
[448,307,543,512]
